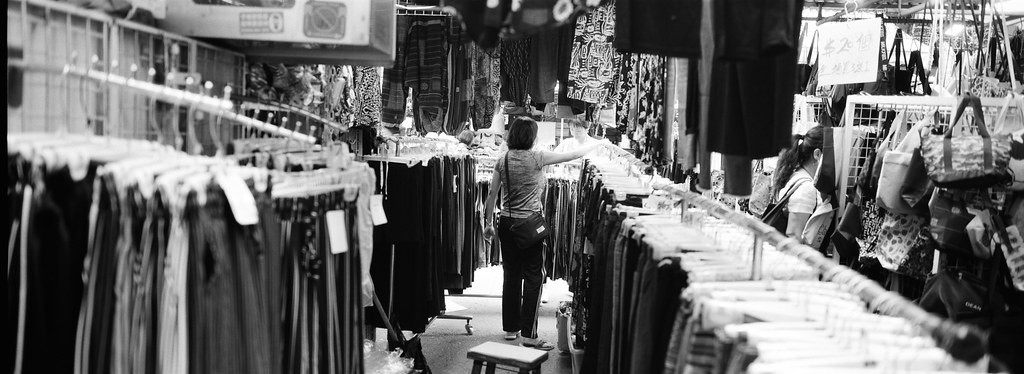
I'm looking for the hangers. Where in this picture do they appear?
[6,56,1007,374]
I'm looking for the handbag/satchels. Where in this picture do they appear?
[798,7,1024,374]
[749,157,771,216]
[510,211,552,251]
[761,176,817,238]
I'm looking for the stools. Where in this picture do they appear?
[466,341,548,374]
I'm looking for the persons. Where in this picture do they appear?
[459,131,475,147]
[771,125,823,242]
[555,119,601,155]
[482,116,611,350]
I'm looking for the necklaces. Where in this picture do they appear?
[801,165,814,179]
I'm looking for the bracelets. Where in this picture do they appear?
[486,219,493,225]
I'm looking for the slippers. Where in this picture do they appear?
[505,330,521,339]
[520,336,553,350]
